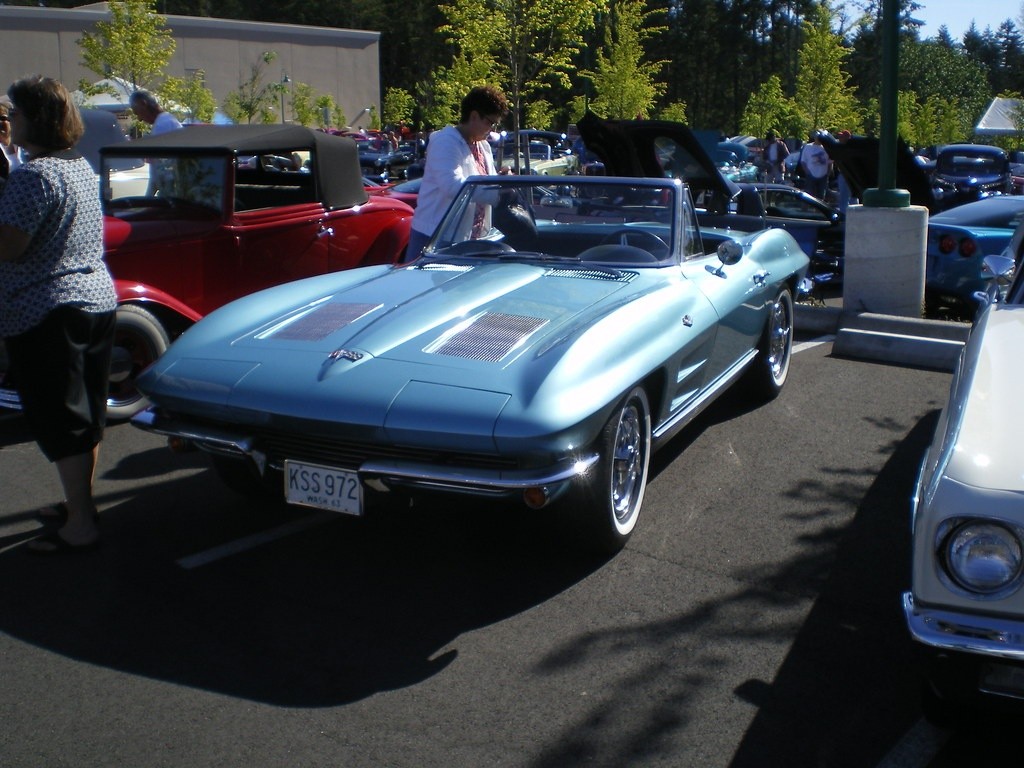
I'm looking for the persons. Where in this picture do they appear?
[763,130,877,213]
[0,75,182,556]
[405,87,515,263]
[358,120,425,151]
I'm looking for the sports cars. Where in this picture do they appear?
[127,175,811,550]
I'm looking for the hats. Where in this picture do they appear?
[837,130,852,139]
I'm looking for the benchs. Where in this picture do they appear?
[526,225,732,259]
[235,184,276,210]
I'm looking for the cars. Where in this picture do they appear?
[898,218,1023,662]
[316,105,1024,317]
[0,123,418,427]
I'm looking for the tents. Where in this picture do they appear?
[69,72,191,114]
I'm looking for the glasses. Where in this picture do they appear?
[479,112,499,129]
[7,108,18,116]
[0,115,8,120]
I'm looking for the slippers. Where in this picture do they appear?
[33,503,99,526]
[27,528,103,557]
